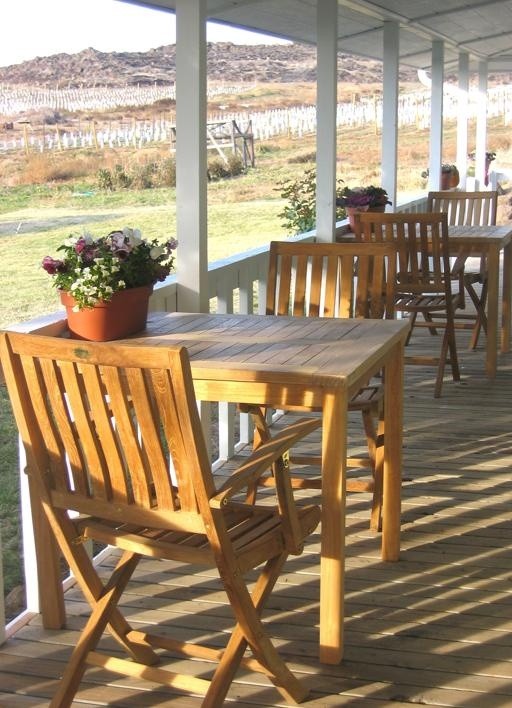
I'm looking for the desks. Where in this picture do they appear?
[24,310,411,665]
[336,225,512,382]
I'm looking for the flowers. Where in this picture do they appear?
[335,185,392,207]
[40,226,177,313]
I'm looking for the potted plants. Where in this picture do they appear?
[468,151,496,187]
[441,164,459,190]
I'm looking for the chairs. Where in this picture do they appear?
[0,328,321,707]
[405,191,497,350]
[234,240,396,534]
[352,212,460,398]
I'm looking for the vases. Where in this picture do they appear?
[53,286,152,341]
